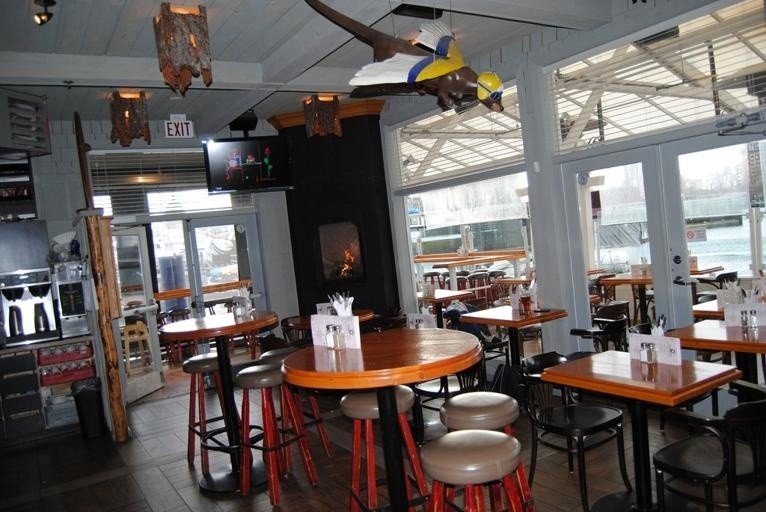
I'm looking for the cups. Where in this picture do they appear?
[37,343,90,377]
[519,295,532,315]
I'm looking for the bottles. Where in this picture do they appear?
[332,325,345,350]
[641,343,648,362]
[233,297,248,316]
[750,309,758,327]
[741,310,749,327]
[647,343,657,363]
[325,324,334,350]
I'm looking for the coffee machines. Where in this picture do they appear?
[0,266,60,347]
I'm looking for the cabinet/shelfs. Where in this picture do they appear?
[1,332,102,454]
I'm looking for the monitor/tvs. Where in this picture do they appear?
[203,133,305,195]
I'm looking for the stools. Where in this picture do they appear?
[121,262,766,511]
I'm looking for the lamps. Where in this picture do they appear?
[106,89,151,148]
[151,1,214,98]
[302,94,343,141]
[31,0,57,26]
